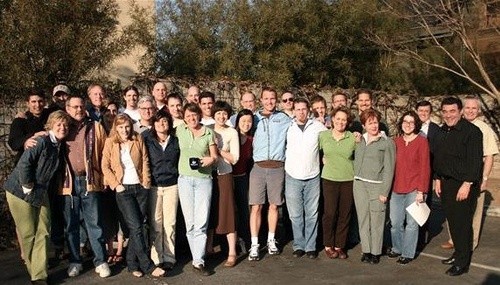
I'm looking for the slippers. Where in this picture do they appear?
[105,254,123,264]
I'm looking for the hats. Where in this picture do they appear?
[53,84,71,95]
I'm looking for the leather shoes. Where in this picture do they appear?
[445,265,468,276]
[441,256,455,264]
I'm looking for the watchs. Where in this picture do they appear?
[481,175,488,181]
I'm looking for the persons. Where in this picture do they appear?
[414,100,440,166]
[324,91,362,134]
[428,98,483,276]
[230,108,254,260]
[172,101,219,276]
[354,107,395,264]
[385,110,431,264]
[284,98,361,258]
[317,109,357,259]
[308,93,336,130]
[441,97,500,249]
[247,84,292,262]
[196,90,218,129]
[4,81,185,285]
[230,90,263,135]
[186,85,202,107]
[278,91,301,119]
[206,100,239,269]
[349,89,390,136]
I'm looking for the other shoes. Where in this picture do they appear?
[325,249,347,259]
[192,264,210,276]
[292,250,317,259]
[129,270,144,277]
[156,263,175,270]
[31,279,49,285]
[149,267,167,278]
[360,253,379,263]
[440,241,453,248]
[56,249,64,260]
[388,251,400,257]
[224,255,237,267]
[397,258,413,265]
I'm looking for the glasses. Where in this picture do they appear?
[68,105,86,110]
[280,98,293,102]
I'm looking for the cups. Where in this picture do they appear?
[189,158,201,170]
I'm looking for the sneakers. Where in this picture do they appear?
[248,244,260,260]
[95,262,111,277]
[67,263,83,277]
[266,238,279,254]
